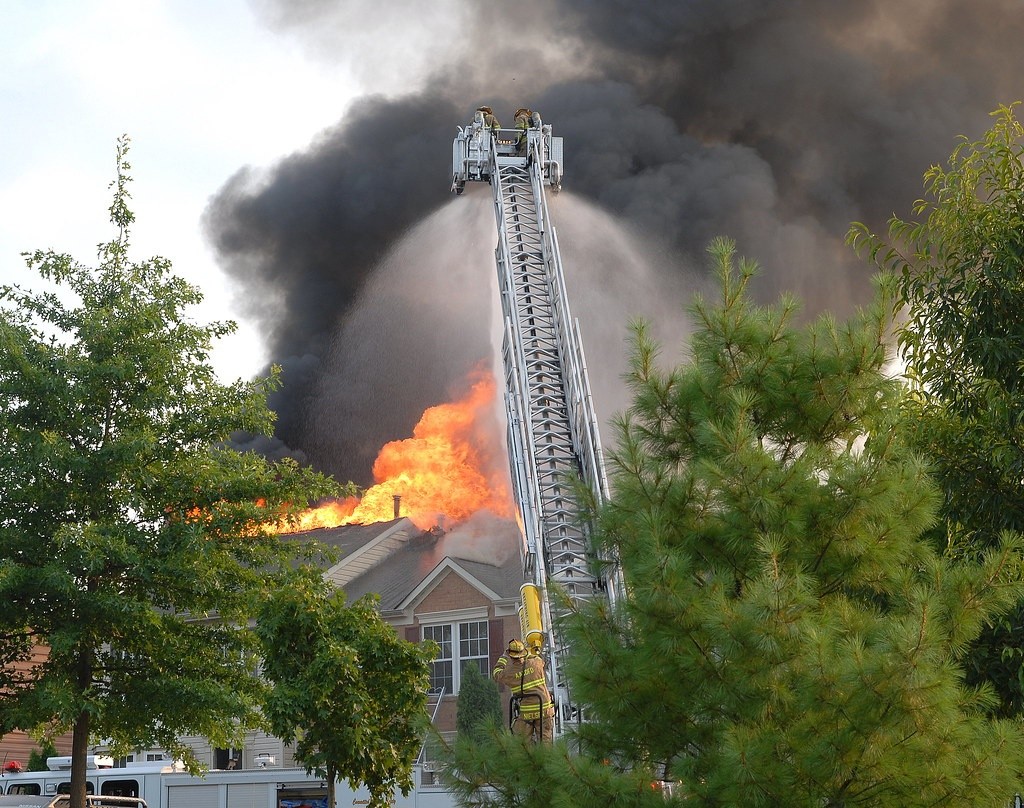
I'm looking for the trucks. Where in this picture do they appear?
[0,791,148,808]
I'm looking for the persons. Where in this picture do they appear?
[468,106,501,141]
[493,639,555,749]
[513,108,534,157]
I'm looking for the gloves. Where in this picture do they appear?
[515,139,521,151]
[503,648,509,658]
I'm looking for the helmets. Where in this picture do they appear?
[509,639,527,658]
[514,109,531,121]
[477,106,492,114]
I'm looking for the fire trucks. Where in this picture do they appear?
[0,111,691,808]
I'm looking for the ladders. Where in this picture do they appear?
[410,686,447,764]
